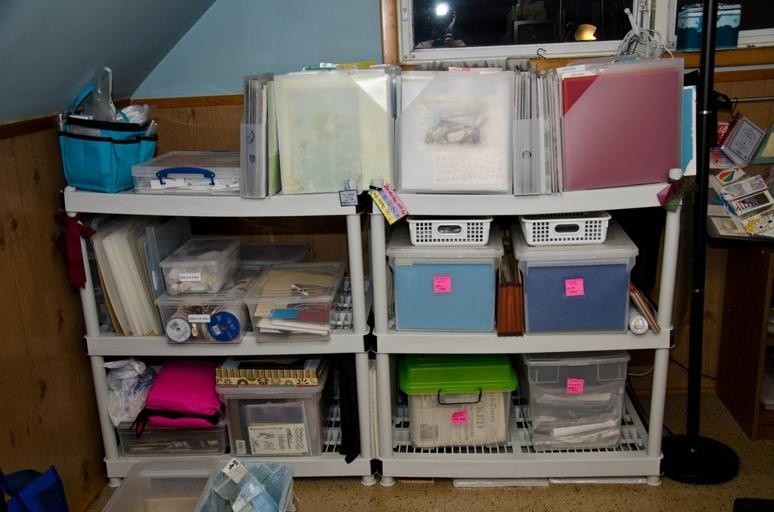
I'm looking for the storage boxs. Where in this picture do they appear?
[509,218,639,336]
[130,149,241,196]
[385,224,505,334]
[100,458,295,512]
[523,350,632,451]
[151,235,348,345]
[215,365,329,458]
[114,411,228,459]
[399,353,520,449]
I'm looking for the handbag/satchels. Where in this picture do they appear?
[56,111,155,191]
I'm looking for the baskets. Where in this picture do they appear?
[520,212,612,246]
[406,216,494,247]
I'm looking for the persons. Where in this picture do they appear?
[411,3,467,50]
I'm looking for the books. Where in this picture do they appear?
[627,281,662,335]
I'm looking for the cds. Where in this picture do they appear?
[166,317,192,342]
[208,312,240,341]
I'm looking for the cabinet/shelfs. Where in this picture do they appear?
[62,183,376,490]
[369,166,685,488]
[709,164,774,443]
